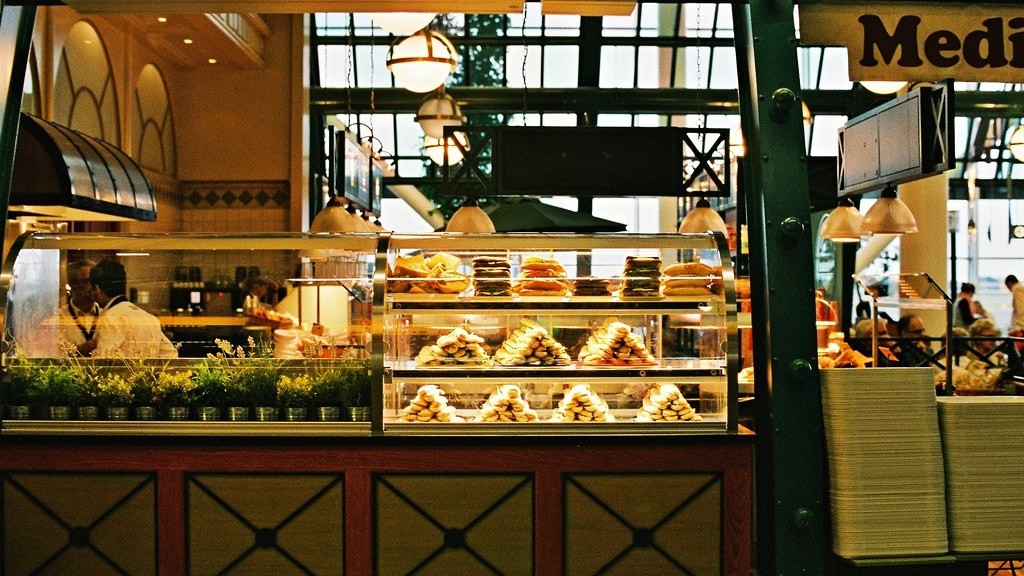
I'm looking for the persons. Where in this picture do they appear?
[31,260,105,358]
[88,258,179,359]
[242,279,268,317]
[855,274,1024,374]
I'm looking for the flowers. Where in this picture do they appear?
[1,324,374,409]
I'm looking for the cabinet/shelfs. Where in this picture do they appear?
[375,231,738,429]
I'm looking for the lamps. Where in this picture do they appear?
[1009,122,1023,164]
[425,131,471,166]
[414,83,467,138]
[678,198,730,239]
[820,196,873,240]
[445,194,496,232]
[345,123,397,171]
[311,196,389,233]
[386,27,459,93]
[859,182,919,234]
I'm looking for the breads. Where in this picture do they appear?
[392,253,713,295]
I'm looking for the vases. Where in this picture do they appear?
[9,405,371,421]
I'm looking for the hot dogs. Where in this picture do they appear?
[395,384,704,422]
[418,318,656,365]
